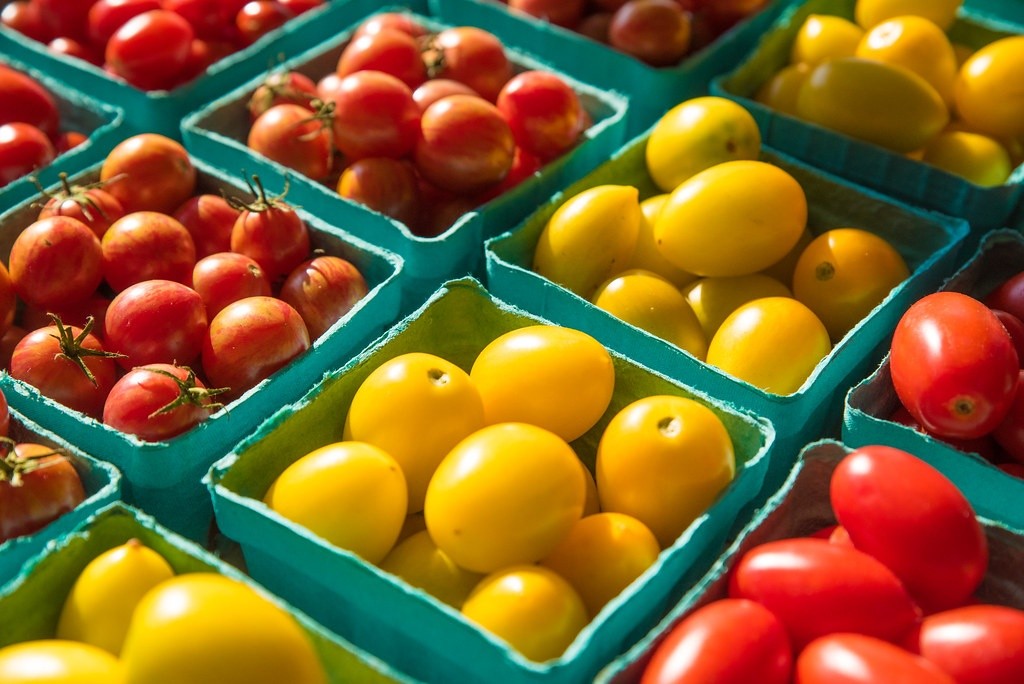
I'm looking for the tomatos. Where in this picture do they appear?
[0,0,1024,684]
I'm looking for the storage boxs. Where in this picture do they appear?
[0,0,388,145]
[425,0,795,142]
[196,269,777,684]
[593,437,1024,684]
[0,51,128,214]
[709,0,1024,267]
[1,159,406,552]
[178,6,632,319]
[482,126,974,499]
[841,223,1023,530]
[0,504,423,684]
